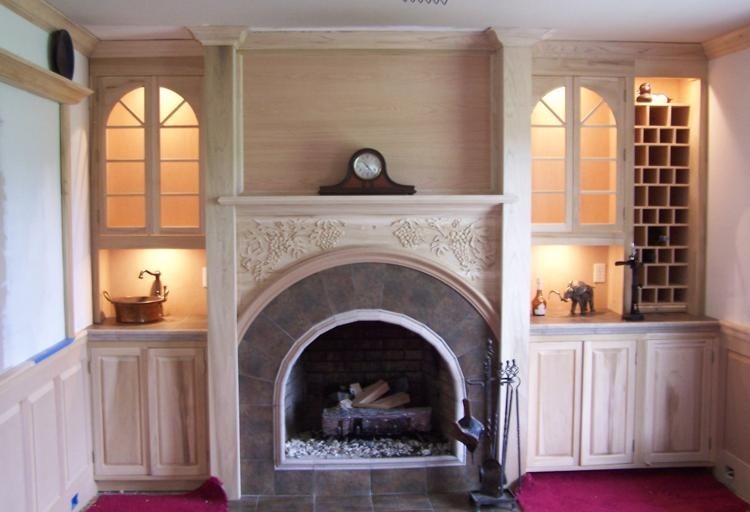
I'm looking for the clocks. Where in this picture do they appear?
[317,147,417,197]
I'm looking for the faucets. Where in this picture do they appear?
[137,269,162,297]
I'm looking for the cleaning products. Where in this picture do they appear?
[533,276,549,316]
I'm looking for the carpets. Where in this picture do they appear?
[78,482,228,511]
[510,469,750,511]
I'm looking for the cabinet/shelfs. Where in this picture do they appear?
[635,103,690,307]
[531,325,637,472]
[91,39,207,249]
[532,43,625,243]
[638,321,721,471]
[84,342,211,493]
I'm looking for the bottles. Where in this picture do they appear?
[650,94,674,104]
[533,278,548,315]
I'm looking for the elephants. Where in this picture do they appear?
[563,280,596,316]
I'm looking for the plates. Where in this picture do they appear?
[52,28,76,82]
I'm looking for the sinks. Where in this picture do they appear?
[103,290,165,322]
